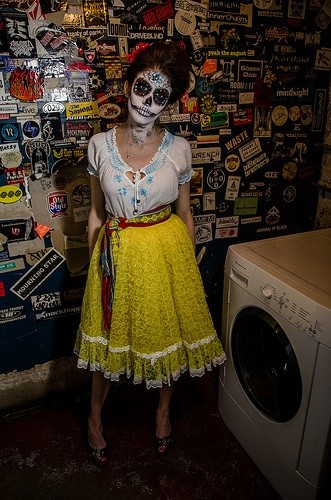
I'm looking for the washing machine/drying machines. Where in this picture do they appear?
[217,227,331,500]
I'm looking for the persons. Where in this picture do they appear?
[71,40,228,469]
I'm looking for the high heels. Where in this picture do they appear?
[87,439,108,466]
[154,431,174,457]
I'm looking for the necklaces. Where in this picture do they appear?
[124,120,136,160]
